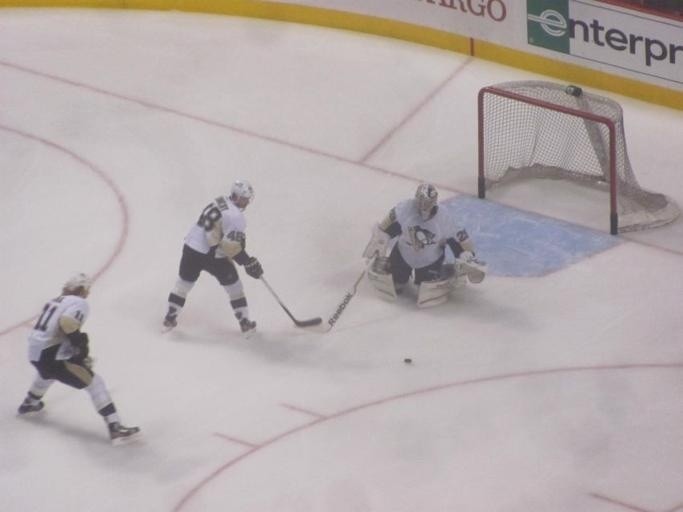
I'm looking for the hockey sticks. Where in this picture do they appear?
[260,276,322,328]
[304,251,378,333]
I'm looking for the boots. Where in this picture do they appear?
[235,311,256,333]
[162,306,178,328]
[17,398,44,415]
[108,421,141,441]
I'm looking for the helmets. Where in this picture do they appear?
[413,181,438,221]
[231,178,254,212]
[64,271,91,299]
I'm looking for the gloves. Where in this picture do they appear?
[244,256,263,279]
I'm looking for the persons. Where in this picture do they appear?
[163,180,263,332]
[18,274,140,439]
[362,184,485,309]
